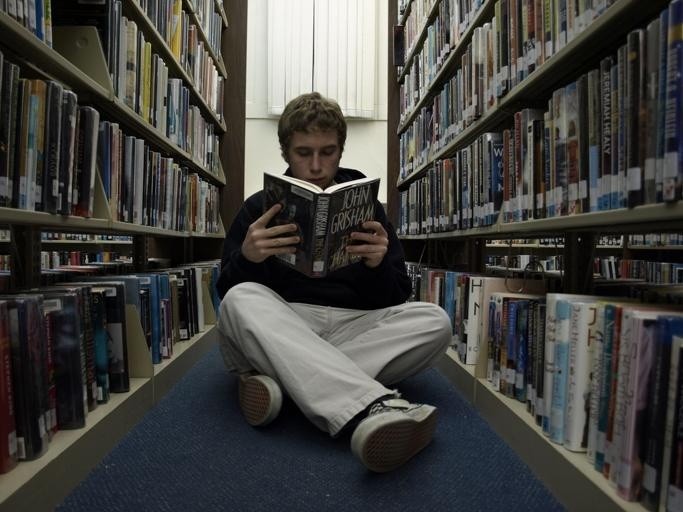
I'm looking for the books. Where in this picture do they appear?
[444,272,682,511]
[395,0,682,304]
[0,0,229,477]
[262,170,381,280]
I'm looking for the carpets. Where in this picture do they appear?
[53,343,568,511]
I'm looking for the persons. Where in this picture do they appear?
[214,89,452,468]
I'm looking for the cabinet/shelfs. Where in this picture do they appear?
[388,0,683,512]
[1,0,246,512]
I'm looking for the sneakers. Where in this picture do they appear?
[234,372,281,431]
[350,395,436,472]
